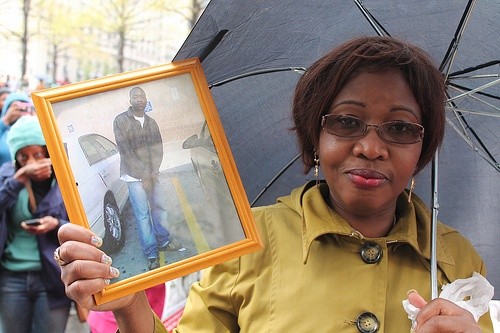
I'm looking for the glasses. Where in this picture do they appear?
[320,114,424,144]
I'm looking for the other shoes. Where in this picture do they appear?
[160,241,182,251]
[148,256,160,270]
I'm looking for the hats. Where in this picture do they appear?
[7,116,46,163]
[2,92,32,117]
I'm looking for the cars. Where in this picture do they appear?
[182,119,235,214]
[62,132,128,249]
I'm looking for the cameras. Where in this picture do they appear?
[17,102,32,113]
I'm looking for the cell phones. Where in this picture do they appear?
[26,218,42,225]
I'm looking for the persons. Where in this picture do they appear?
[114,86,182,269]
[0,86,188,333]
[53,36,494,333]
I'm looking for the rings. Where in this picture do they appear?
[54,246,66,265]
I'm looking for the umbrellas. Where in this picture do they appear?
[172,1,499,304]
